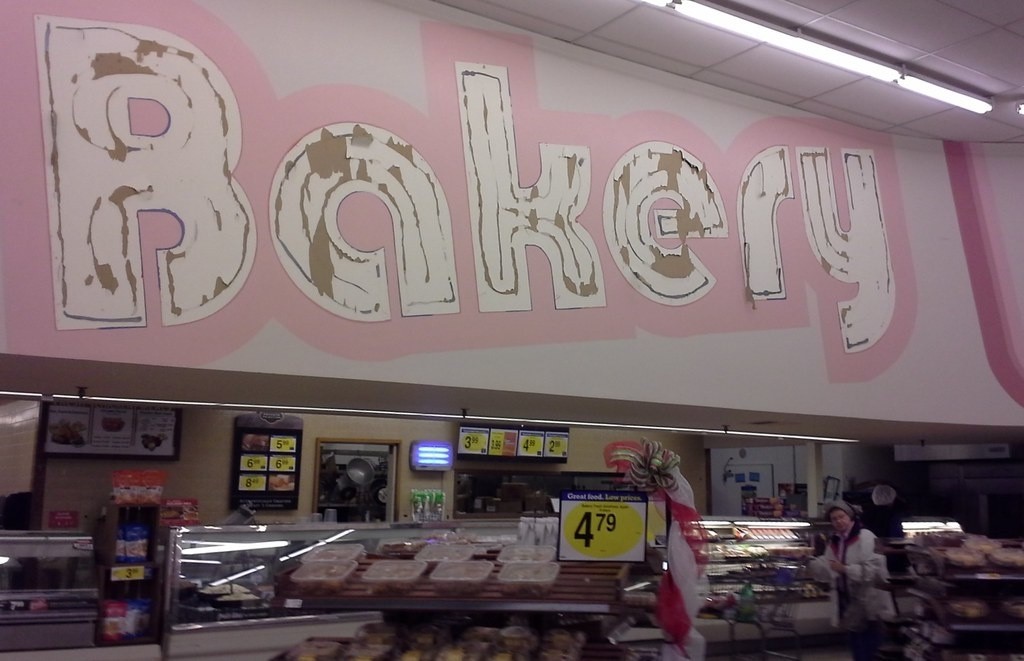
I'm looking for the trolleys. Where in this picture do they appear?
[698,554,816,660]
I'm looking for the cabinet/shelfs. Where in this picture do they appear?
[0,516,666,661]
[691,517,838,643]
[874,531,1024,661]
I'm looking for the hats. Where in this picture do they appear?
[823,500,854,518]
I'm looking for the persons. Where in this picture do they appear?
[796,500,896,661]
[861,485,922,581]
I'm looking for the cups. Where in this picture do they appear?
[298,508,337,523]
[412,490,446,523]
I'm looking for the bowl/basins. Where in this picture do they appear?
[346,458,374,486]
